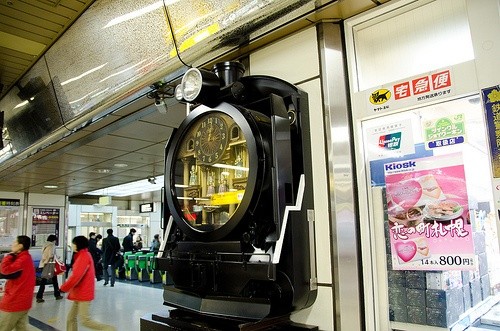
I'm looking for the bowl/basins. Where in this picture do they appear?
[425,200,461,219]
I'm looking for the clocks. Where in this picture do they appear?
[193,115,229,165]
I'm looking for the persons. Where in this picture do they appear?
[151,234,161,255]
[36,234,63,303]
[57,236,118,331]
[93,234,102,258]
[89,233,102,281]
[119,228,138,280]
[0,235,36,331]
[101,229,120,287]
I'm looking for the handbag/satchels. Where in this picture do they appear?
[112,254,124,268]
[41,255,66,279]
[0,255,21,279]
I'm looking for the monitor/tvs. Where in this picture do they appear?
[139,203,153,213]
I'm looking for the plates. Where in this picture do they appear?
[422,207,464,221]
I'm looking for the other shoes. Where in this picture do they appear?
[55,296,64,300]
[36,298,45,302]
